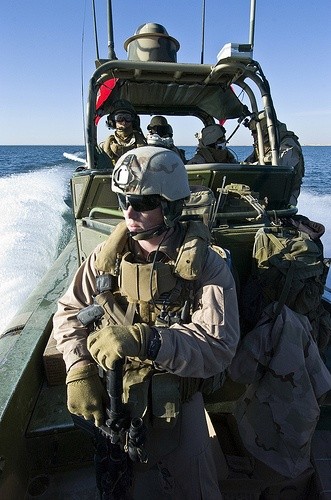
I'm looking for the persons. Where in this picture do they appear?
[95,100,306,206]
[52,146,239,500]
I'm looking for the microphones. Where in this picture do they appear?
[126,223,164,238]
[116,127,128,131]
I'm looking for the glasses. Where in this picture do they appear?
[116,114,132,123]
[117,193,161,212]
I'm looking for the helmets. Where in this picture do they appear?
[242,110,286,134]
[110,99,136,114]
[111,146,191,202]
[147,116,172,129]
[201,124,226,147]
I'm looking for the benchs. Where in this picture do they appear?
[24,379,331,467]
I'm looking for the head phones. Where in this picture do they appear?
[161,198,183,227]
[107,114,140,130]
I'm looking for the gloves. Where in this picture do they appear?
[65,364,108,427]
[86,323,150,372]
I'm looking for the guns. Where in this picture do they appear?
[93,356,149,500]
